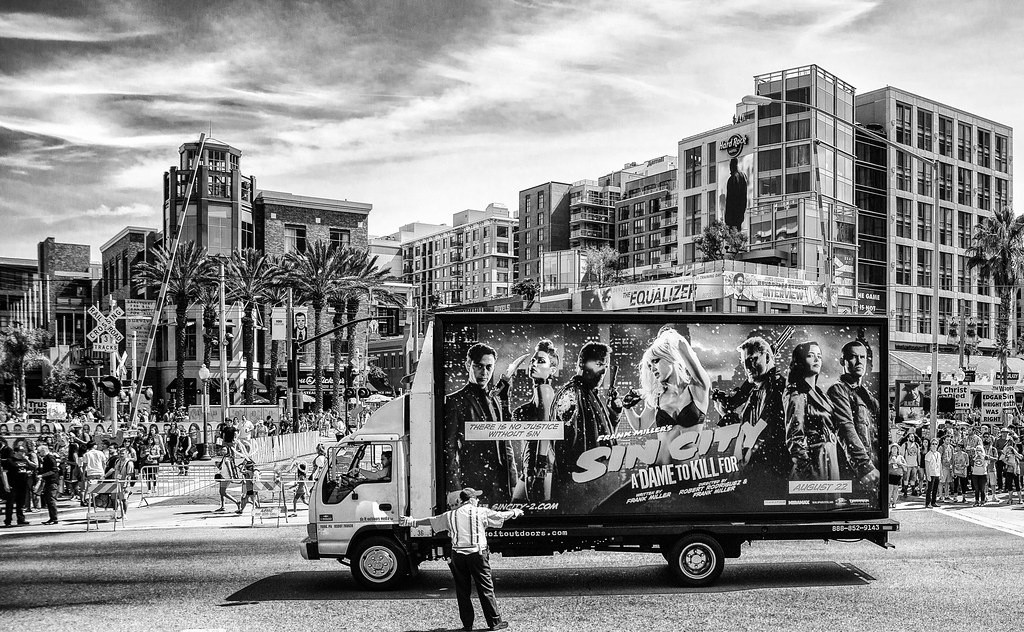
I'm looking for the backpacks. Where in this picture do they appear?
[73,465,83,482]
[125,459,136,487]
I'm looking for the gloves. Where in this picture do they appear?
[398,514,419,529]
[510,508,525,520]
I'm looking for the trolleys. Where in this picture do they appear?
[87,481,126,519]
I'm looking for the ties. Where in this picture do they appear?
[486,395,503,467]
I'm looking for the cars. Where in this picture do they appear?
[903,418,974,436]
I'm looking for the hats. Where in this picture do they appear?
[460,488,482,504]
[1000,428,1009,432]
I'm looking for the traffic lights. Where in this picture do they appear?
[345,387,370,398]
[96,376,121,398]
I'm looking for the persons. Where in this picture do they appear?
[207,423,213,443]
[900,383,923,406]
[164,424,171,434]
[349,451,392,480]
[950,317,958,337]
[888,403,1024,509]
[967,318,977,338]
[399,488,525,631]
[725,273,749,300]
[719,189,725,223]
[188,423,201,444]
[820,284,827,303]
[445,329,880,507]
[294,312,307,340]
[724,158,748,234]
[0,398,374,527]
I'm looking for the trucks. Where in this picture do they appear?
[299,312,900,590]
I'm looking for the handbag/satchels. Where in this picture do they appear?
[214,474,222,482]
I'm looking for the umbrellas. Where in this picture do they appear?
[366,394,394,408]
[303,395,316,402]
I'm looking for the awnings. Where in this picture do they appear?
[165,378,197,394]
[890,351,1024,394]
[266,375,378,395]
[210,378,237,393]
[244,379,267,392]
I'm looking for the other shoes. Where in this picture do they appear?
[939,496,944,503]
[147,490,151,495]
[985,497,988,503]
[999,489,1002,494]
[461,625,473,631]
[17,521,30,526]
[973,500,985,507]
[962,497,966,503]
[1007,499,1013,504]
[945,496,952,501]
[178,471,184,476]
[992,494,1000,502]
[288,512,297,517]
[185,471,189,476]
[236,500,241,509]
[954,497,958,502]
[888,503,896,508]
[41,519,58,525]
[80,500,88,506]
[926,502,941,509]
[116,514,128,521]
[215,506,225,512]
[31,507,40,513]
[153,485,157,491]
[490,621,509,631]
[5,524,11,527]
[235,510,243,515]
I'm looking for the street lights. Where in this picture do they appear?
[292,316,395,433]
[199,363,211,460]
